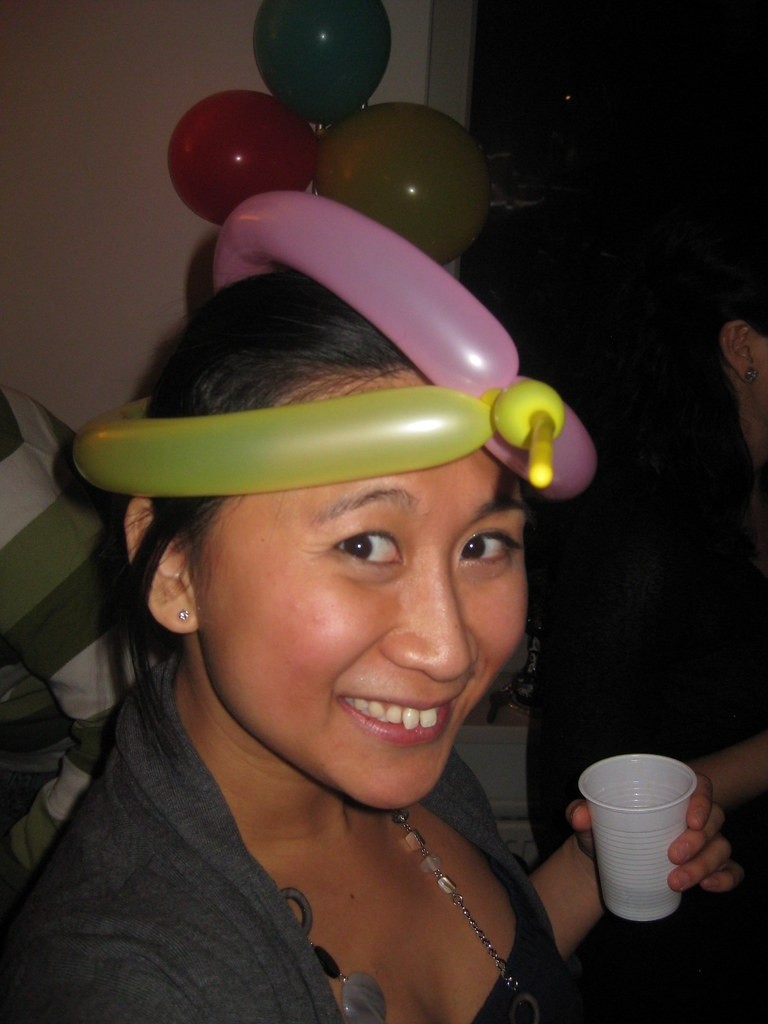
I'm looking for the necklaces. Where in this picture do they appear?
[277,803,543,1024]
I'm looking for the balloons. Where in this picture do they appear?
[70,191,597,498]
[314,103,489,267]
[167,89,321,226]
[253,0,392,124]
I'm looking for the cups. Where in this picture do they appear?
[578,754,697,922]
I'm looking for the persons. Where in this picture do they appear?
[1,376,160,908]
[526,235,768,1024]
[0,256,743,1024]
